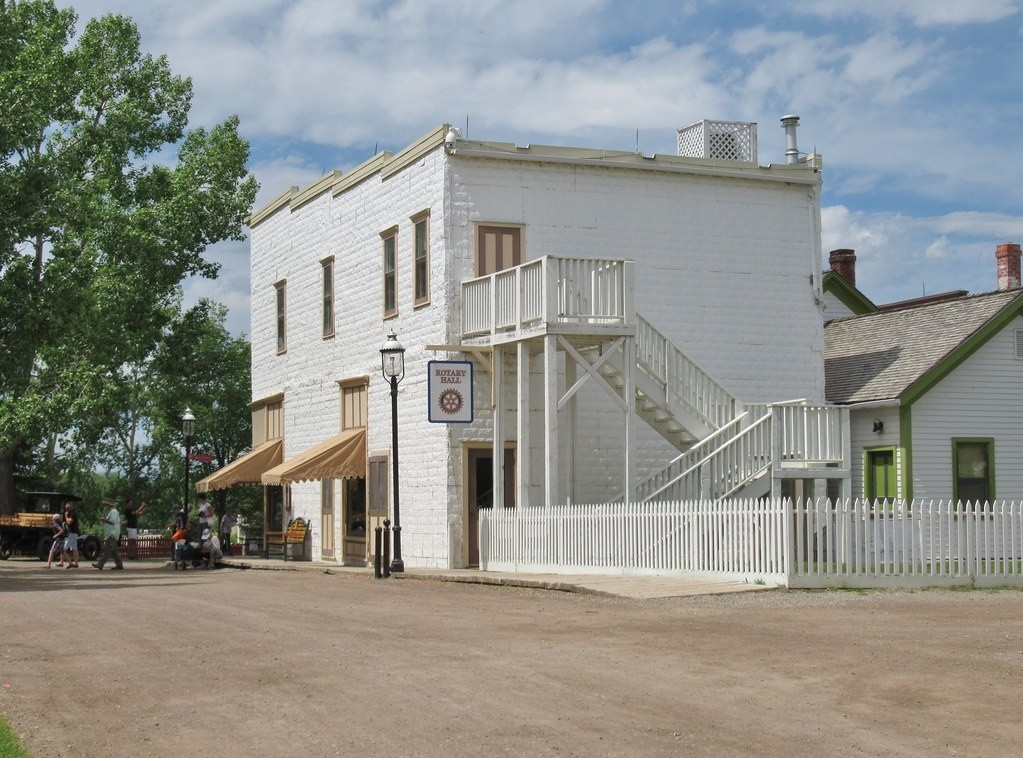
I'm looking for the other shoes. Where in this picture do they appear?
[127,556,135,560]
[65,564,73,570]
[199,565,206,570]
[41,566,50,569]
[73,565,78,568]
[56,564,63,567]
[206,565,214,569]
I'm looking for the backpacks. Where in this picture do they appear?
[53,521,69,538]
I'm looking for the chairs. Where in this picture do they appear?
[265,518,311,562]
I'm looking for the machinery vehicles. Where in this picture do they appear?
[0,491,99,561]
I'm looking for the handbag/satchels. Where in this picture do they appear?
[172,529,184,541]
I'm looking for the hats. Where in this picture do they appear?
[103,498,115,506]
[53,514,60,520]
[169,504,179,513]
[201,529,211,540]
[202,542,212,552]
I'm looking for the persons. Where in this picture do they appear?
[41,501,80,569]
[220,508,238,556]
[166,492,223,570]
[92,499,124,570]
[124,500,146,561]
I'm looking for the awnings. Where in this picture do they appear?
[261,428,366,485]
[196,438,283,492]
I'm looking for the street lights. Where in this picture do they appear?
[181,406,195,529]
[378,328,406,572]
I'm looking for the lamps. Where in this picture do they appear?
[873,418,883,432]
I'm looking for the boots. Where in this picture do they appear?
[111,562,122,570]
[92,559,106,570]
